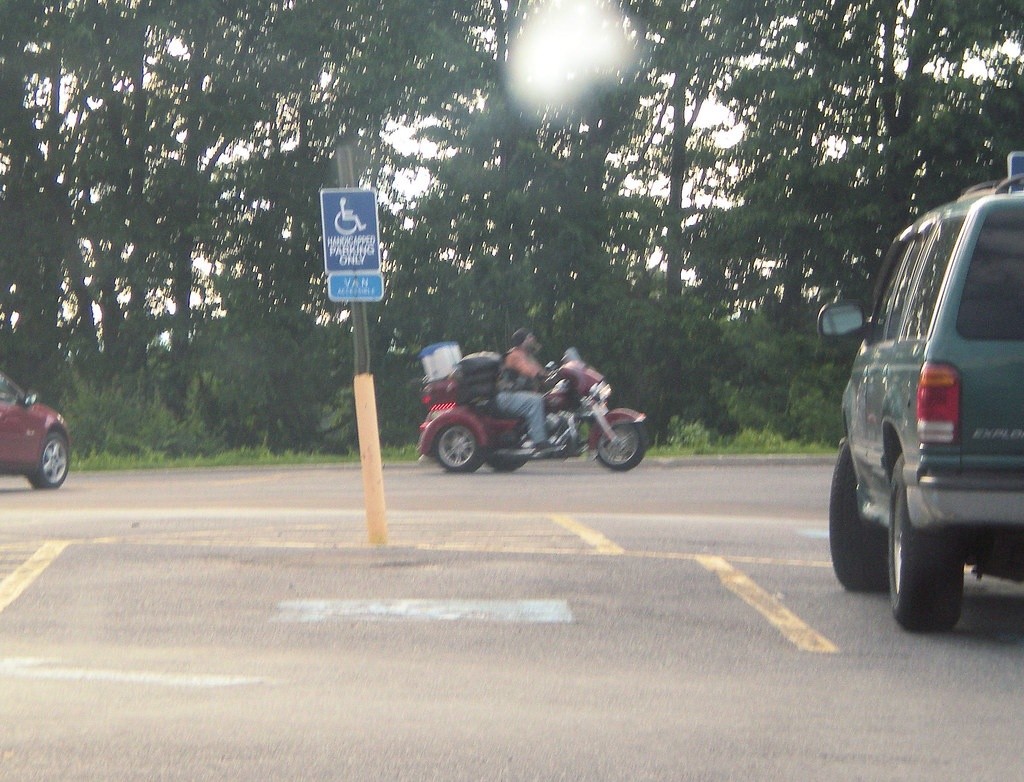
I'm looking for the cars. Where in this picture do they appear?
[0,378,71,491]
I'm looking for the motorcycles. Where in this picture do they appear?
[415,348,651,477]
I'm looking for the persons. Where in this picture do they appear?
[494,327,559,451]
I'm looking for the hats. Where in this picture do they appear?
[511,328,530,346]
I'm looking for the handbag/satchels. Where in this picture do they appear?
[456,350,501,406]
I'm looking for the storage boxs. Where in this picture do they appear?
[419,341,462,380]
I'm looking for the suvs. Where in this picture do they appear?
[814,170,1023,637]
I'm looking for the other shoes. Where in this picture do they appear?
[535,440,554,451]
